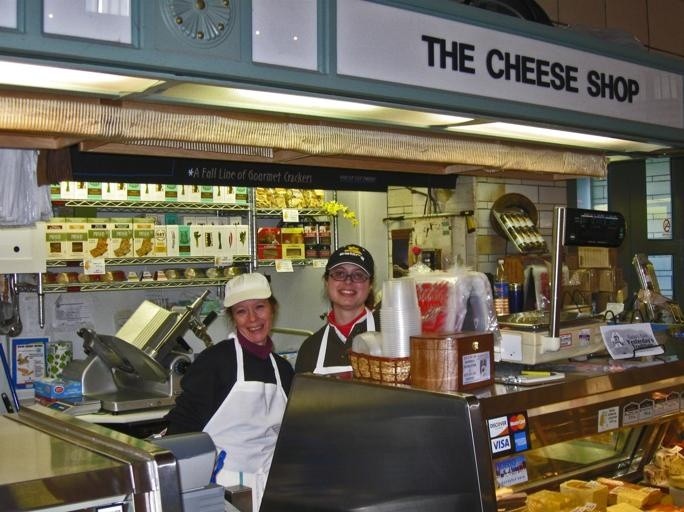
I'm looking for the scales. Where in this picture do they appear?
[497,205,627,365]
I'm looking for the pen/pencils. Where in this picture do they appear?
[519,370,557,376]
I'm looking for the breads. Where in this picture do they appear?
[495,446,684,512]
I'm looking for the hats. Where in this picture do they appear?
[326,244,374,277]
[223,272,272,308]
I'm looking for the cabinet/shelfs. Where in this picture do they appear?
[41,180,340,289]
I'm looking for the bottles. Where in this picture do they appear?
[500,212,547,251]
[491,260,509,315]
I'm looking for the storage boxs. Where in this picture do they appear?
[409,330,494,392]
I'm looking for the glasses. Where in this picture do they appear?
[326,271,369,283]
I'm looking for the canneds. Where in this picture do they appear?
[282,221,333,258]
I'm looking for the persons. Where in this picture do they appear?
[161,271,294,512]
[294,243,382,374]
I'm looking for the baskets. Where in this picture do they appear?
[346,347,411,385]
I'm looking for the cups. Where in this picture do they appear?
[353,277,422,359]
[510,283,524,314]
